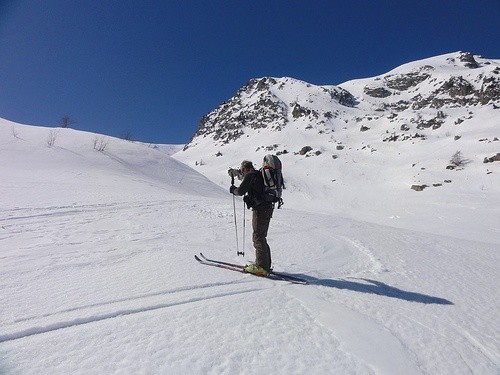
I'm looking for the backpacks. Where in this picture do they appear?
[248,154,286,209]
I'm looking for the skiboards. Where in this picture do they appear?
[194,251,310,286]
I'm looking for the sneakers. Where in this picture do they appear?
[247,266,270,276]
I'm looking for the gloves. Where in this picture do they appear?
[230,185,237,195]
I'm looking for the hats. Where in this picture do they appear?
[240,160,251,173]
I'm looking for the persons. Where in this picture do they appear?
[229,160,276,278]
